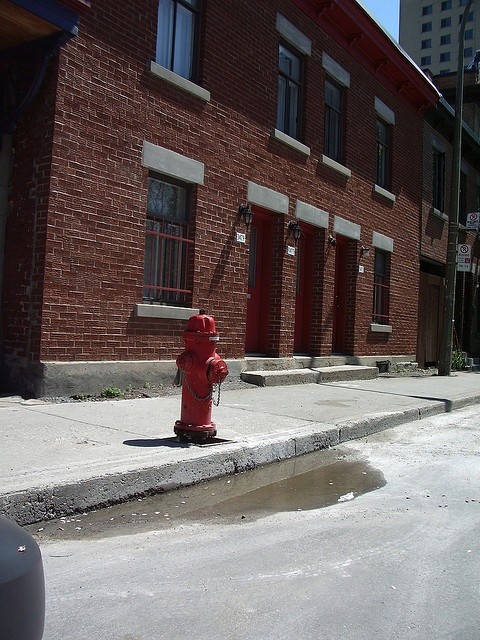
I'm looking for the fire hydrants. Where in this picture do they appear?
[174,310,229,438]
